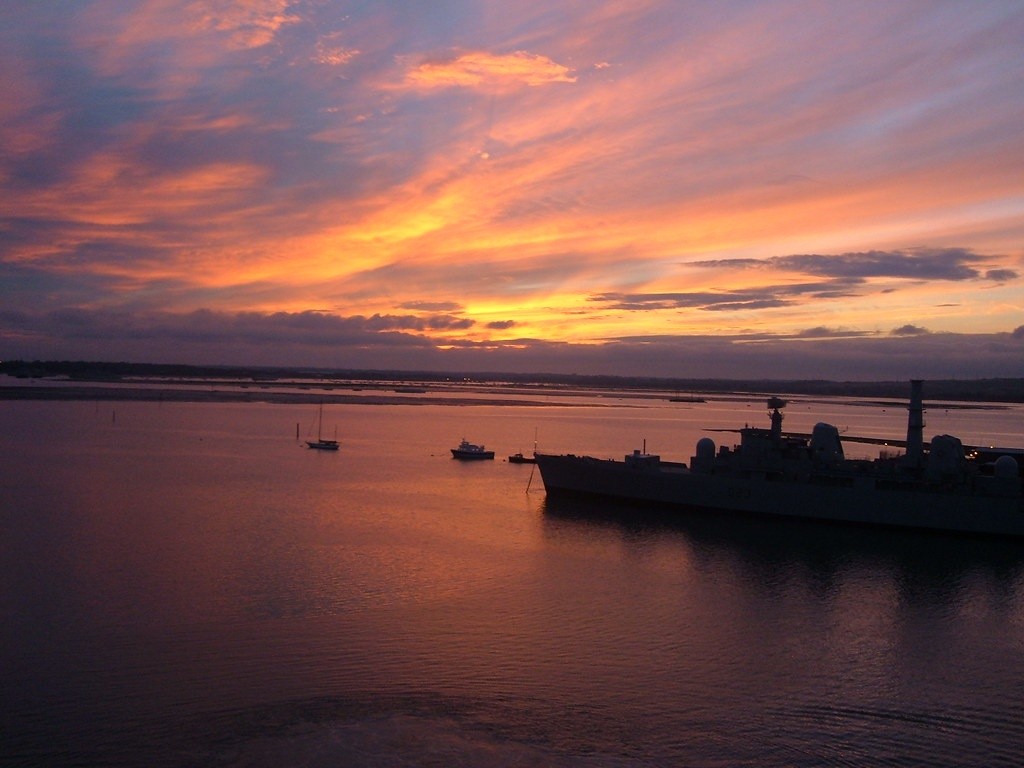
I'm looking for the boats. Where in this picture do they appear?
[668,393,706,403]
[507,452,536,464]
[392,388,427,393]
[449,437,496,461]
[533,378,1023,539]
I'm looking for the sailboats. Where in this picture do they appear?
[304,401,342,451]
